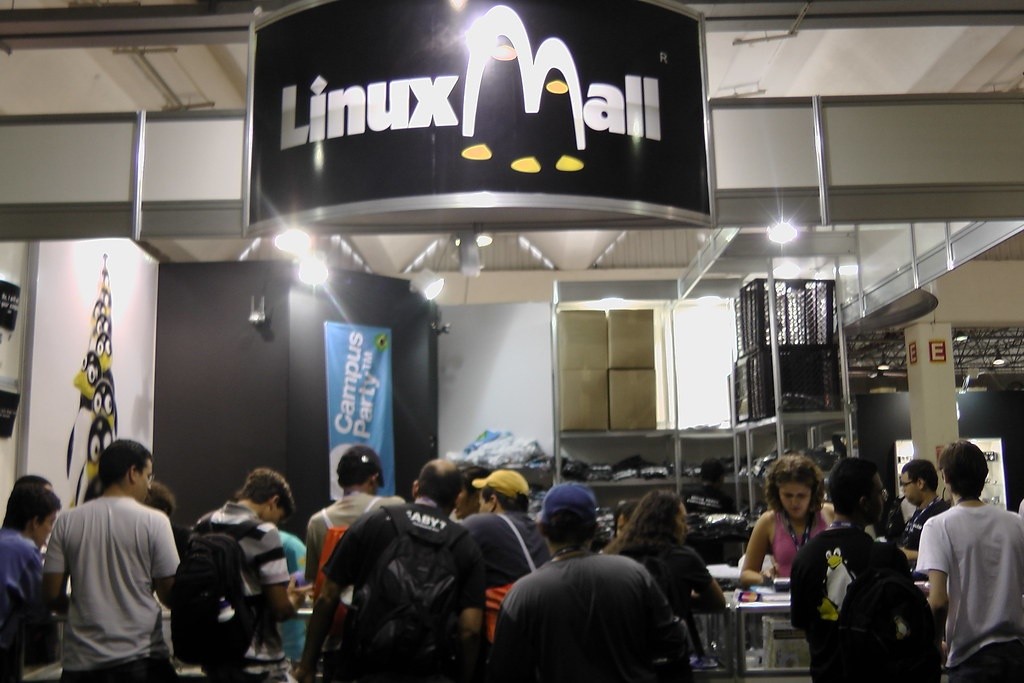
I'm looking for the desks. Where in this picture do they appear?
[678,586,812,683]
[15,607,312,683]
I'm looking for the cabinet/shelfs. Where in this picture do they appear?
[728,257,852,559]
[550,300,747,563]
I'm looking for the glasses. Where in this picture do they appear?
[900,479,916,488]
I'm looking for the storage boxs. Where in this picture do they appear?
[729,278,842,421]
[556,307,657,433]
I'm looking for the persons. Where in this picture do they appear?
[914,444,1024,679]
[494,481,698,682]
[0,470,66,653]
[298,444,486,683]
[170,468,314,682]
[78,468,177,520]
[599,491,730,616]
[788,459,942,683]
[682,460,742,528]
[741,456,832,585]
[456,465,550,645]
[41,442,179,682]
[896,460,948,562]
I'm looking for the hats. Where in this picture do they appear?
[471,469,529,500]
[542,482,595,524]
[338,446,385,488]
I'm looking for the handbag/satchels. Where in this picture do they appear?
[483,513,537,645]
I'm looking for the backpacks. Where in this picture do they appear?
[313,506,352,636]
[170,512,267,664]
[836,542,934,683]
[347,505,469,683]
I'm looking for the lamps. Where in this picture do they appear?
[993,348,1004,365]
[877,351,890,372]
[954,331,969,344]
